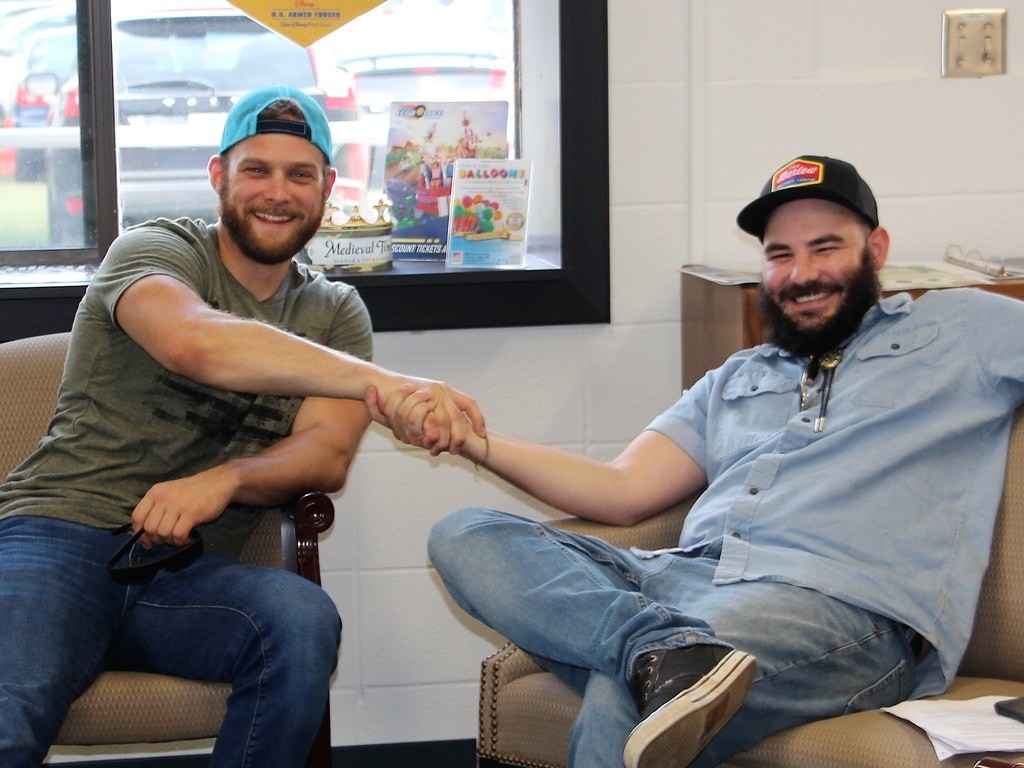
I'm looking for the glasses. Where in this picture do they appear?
[106,523,203,586]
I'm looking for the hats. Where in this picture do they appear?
[737,155,878,244]
[219,86,333,166]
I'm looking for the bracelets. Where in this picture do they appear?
[481,436,489,466]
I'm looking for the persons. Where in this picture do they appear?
[358,154,1024,768]
[0,87,486,768]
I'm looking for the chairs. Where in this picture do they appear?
[0,332,336,768]
[478,408,1024,768]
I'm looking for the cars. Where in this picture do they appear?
[7,6,514,234]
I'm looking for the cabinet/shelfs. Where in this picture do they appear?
[679,263,1023,402]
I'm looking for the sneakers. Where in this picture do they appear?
[621,643,756,768]
